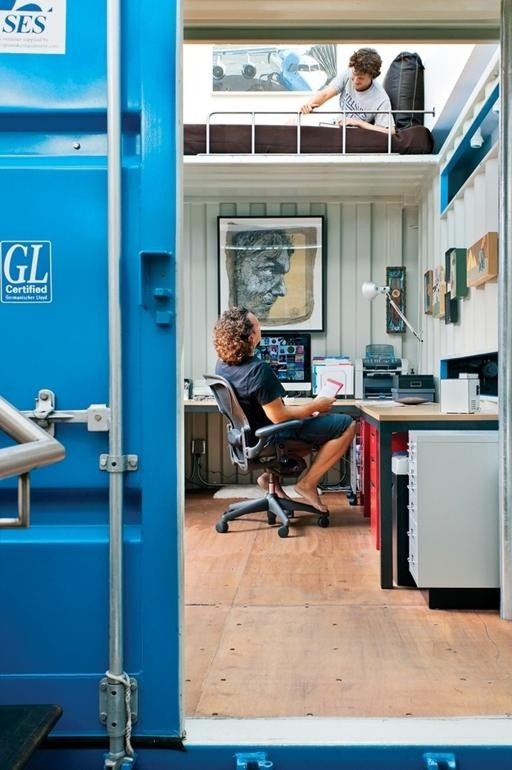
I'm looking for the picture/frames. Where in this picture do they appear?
[210,44,339,96]
[446,247,454,281]
[449,248,467,300]
[385,266,406,333]
[466,232,497,288]
[433,281,446,319]
[424,270,434,314]
[254,333,311,382]
[444,292,457,325]
[217,214,325,333]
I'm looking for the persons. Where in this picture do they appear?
[228,230,294,322]
[298,48,397,136]
[214,306,357,513]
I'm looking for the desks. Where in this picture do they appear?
[186,395,499,589]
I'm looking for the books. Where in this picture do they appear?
[311,379,343,418]
[312,356,354,396]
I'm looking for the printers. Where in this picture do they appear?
[354,343,408,398]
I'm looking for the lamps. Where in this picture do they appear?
[362,280,423,343]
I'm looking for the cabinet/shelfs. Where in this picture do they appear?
[406,430,500,610]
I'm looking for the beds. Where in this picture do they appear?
[183,52,439,193]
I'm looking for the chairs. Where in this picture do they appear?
[203,373,330,538]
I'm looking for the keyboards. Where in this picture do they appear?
[283,395,312,405]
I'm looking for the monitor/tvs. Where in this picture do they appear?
[259,333,312,392]
